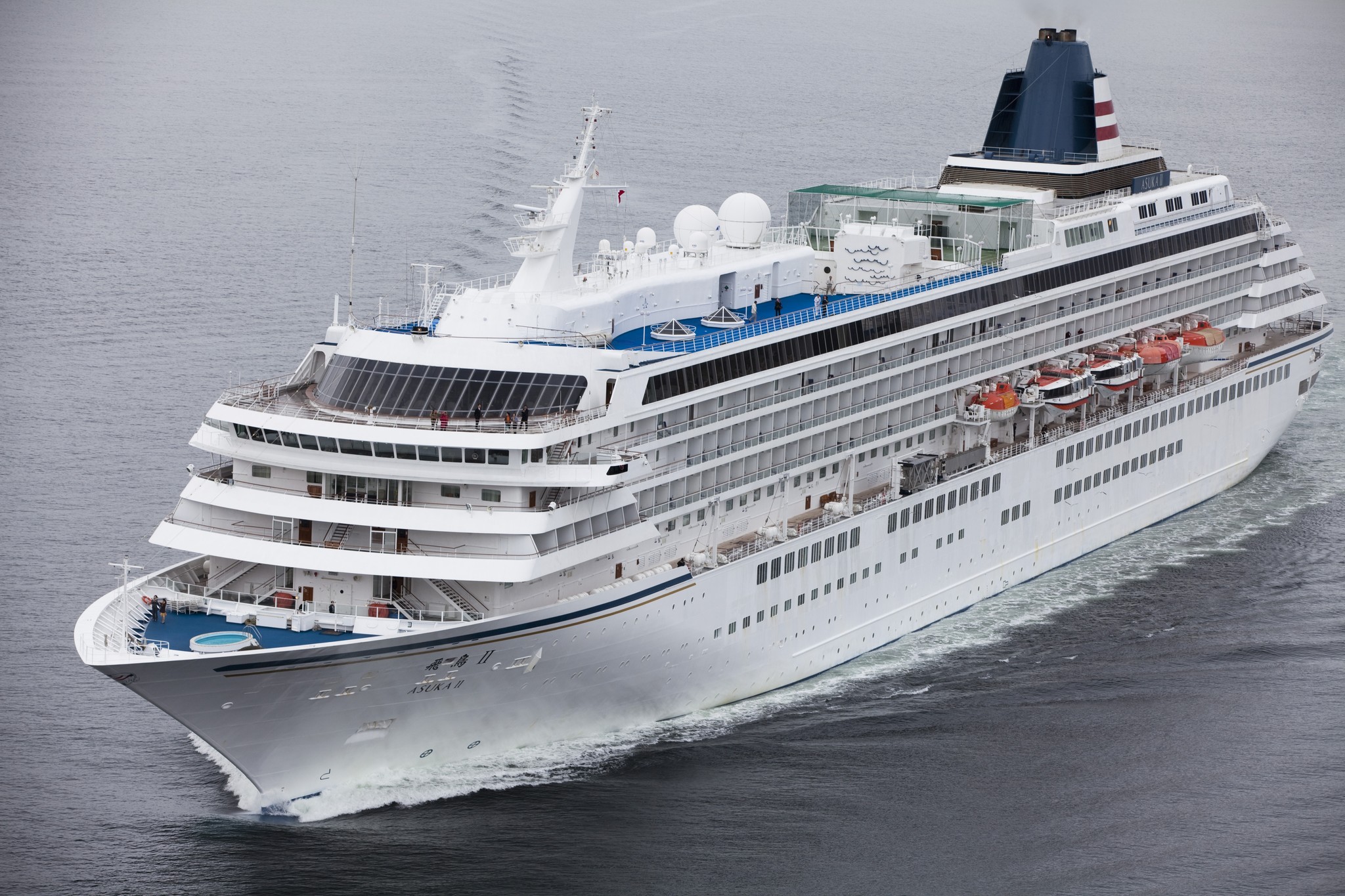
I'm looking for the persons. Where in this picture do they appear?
[299,601,307,612]
[473,403,483,430]
[1077,328,1084,342]
[911,347,915,363]
[430,409,444,430]
[935,404,941,420]
[1119,287,1125,300]
[504,412,513,433]
[821,295,829,316]
[747,301,757,323]
[511,413,519,434]
[826,276,834,295]
[518,405,529,432]
[329,600,335,613]
[814,294,821,315]
[439,410,449,431]
[948,368,953,384]
[158,598,167,624]
[1065,332,1071,346]
[1012,422,1017,441]
[1041,424,1048,444]
[676,558,685,568]
[151,595,161,622]
[774,298,781,317]
[1115,289,1120,301]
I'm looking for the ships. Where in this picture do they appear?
[73,29,1335,823]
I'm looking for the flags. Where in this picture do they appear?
[617,190,625,206]
